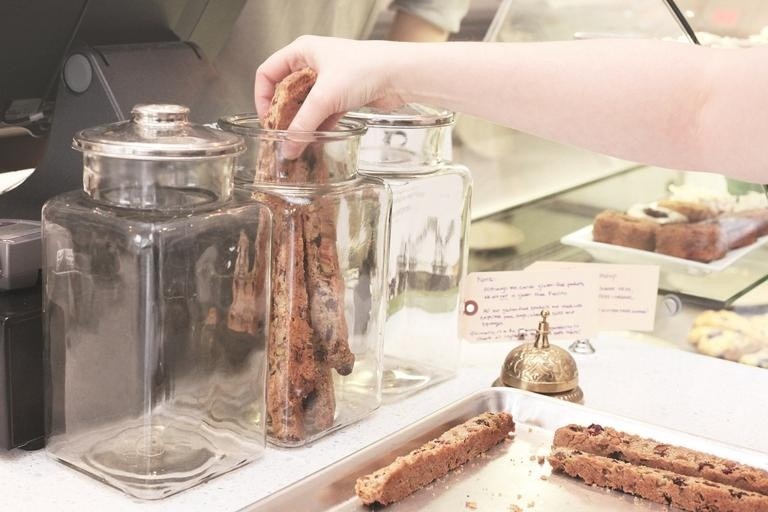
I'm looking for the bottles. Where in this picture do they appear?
[305,100,474,402]
[30,100,268,499]
[212,112,386,450]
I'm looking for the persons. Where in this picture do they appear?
[252,33,768,188]
[211,0,470,113]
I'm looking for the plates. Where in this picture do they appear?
[558,224,768,278]
[467,216,527,252]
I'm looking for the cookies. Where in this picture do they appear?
[355,411,516,505]
[546,422,768,512]
[226,66,356,445]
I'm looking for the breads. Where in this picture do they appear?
[594,178,768,370]
